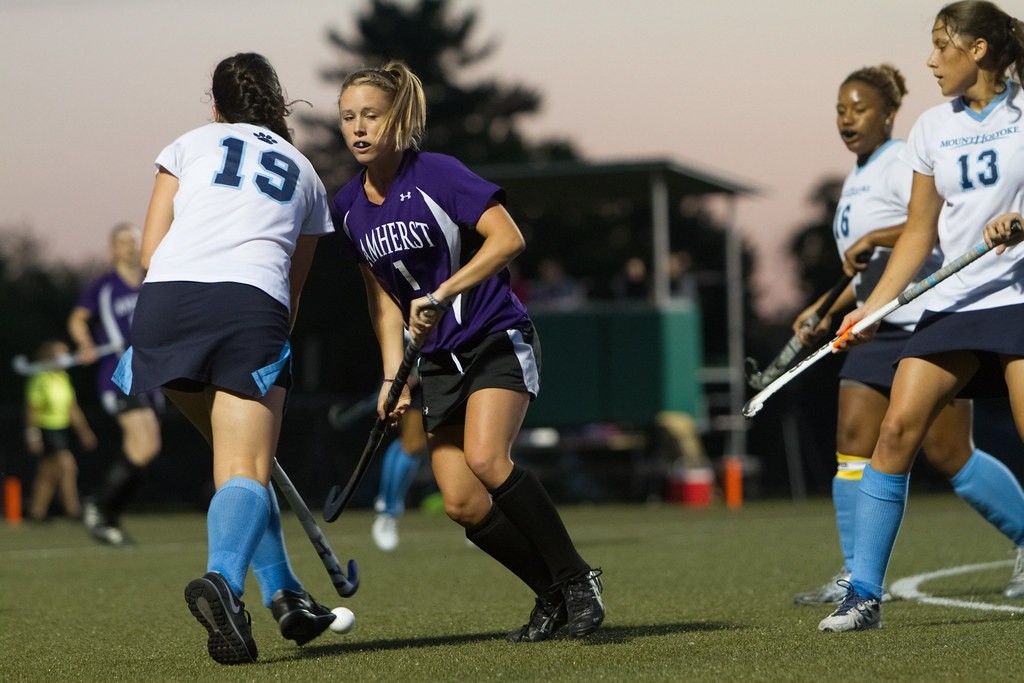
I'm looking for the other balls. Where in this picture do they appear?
[329,606,355,635]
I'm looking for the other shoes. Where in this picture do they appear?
[81,494,124,546]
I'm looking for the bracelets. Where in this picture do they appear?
[426,293,449,311]
[383,379,408,384]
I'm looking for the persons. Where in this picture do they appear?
[329,62,605,643]
[372,408,479,551]
[790,0,1024,631]
[110,52,333,662]
[70,223,166,550]
[24,340,98,522]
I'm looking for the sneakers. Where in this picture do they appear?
[817,579,881,633]
[183,571,258,663]
[272,588,337,646]
[369,510,401,552]
[1001,544,1024,600]
[507,591,566,642]
[794,566,893,604]
[559,566,606,642]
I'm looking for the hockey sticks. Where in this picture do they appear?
[12,335,131,376]
[741,218,1023,420]
[271,455,360,599]
[742,253,872,391]
[322,306,438,524]
